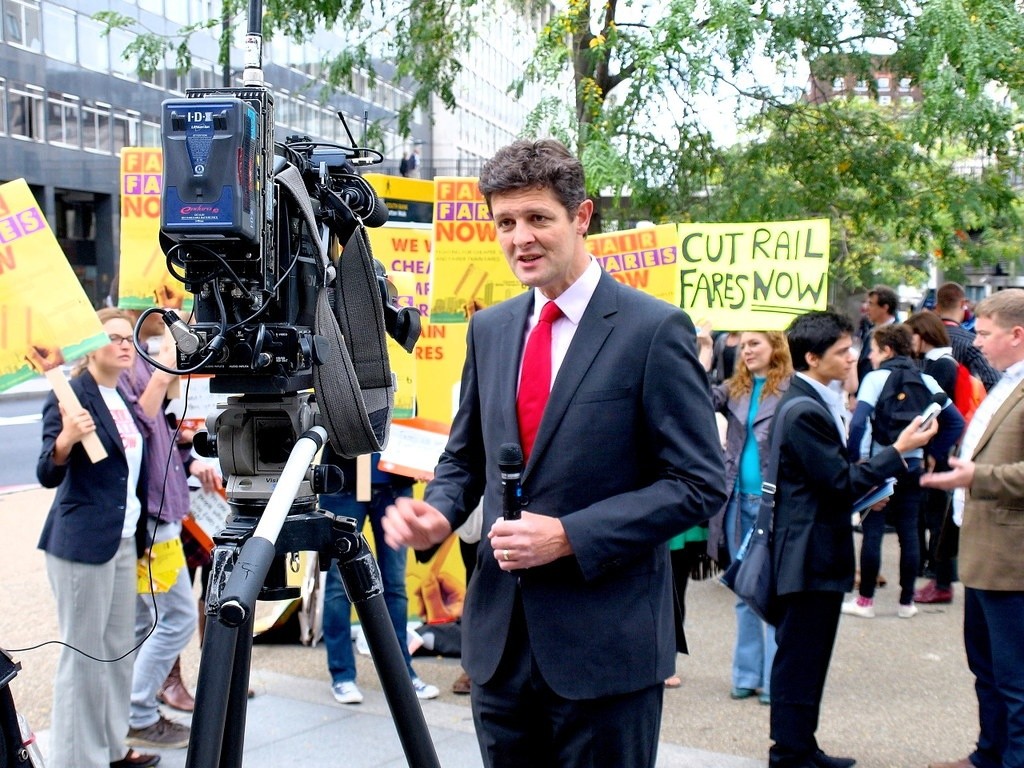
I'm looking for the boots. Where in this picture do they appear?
[156,655,193,712]
[198,599,254,699]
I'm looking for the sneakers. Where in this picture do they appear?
[413,678,440,700]
[126,716,190,750]
[332,681,363,704]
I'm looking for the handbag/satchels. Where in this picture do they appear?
[731,529,775,627]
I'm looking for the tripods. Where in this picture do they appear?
[185,370,442,768]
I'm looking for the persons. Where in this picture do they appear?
[452,383,483,694]
[706,329,795,705]
[696,330,744,454]
[379,137,729,768]
[663,518,709,689]
[318,441,439,704]
[919,288,1024,768]
[827,281,1002,618]
[765,311,938,768]
[36,274,222,768]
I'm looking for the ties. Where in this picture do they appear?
[515,302,563,466]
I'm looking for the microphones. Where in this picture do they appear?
[497,442,525,576]
[918,392,947,429]
[341,187,389,228]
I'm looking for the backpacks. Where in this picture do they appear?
[947,355,987,447]
[871,368,934,447]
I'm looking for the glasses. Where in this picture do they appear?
[109,334,134,345]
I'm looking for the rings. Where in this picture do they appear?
[504,550,509,560]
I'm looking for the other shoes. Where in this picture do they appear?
[914,582,952,604]
[818,748,855,768]
[760,692,771,705]
[899,602,918,618]
[841,598,874,618]
[730,687,754,698]
[110,748,159,768]
[452,672,470,695]
[928,758,976,768]
